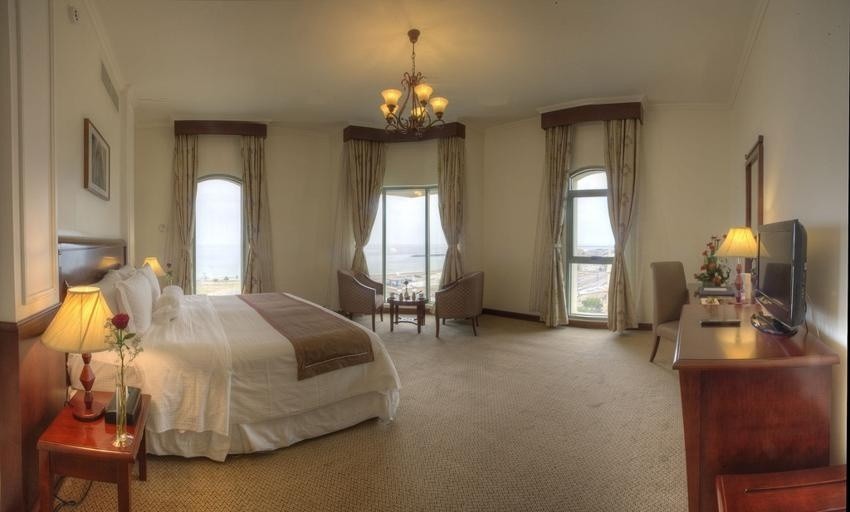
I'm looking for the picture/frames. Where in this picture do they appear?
[84,118,111,201]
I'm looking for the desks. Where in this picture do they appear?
[714,465,848,512]
[36,391,152,511]
[387,296,428,333]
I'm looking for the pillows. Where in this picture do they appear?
[94,263,185,336]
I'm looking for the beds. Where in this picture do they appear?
[58,236,402,463]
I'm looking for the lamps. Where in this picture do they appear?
[714,226,757,304]
[143,257,166,277]
[39,285,126,422]
[380,29,449,139]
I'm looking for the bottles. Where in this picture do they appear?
[742,272,752,305]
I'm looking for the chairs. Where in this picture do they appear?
[435,271,484,336]
[649,261,686,361]
[337,267,384,332]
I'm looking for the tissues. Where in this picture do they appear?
[698,285,736,300]
[104,383,142,424]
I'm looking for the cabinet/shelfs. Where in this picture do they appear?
[672,282,840,512]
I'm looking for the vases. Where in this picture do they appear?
[110,383,133,447]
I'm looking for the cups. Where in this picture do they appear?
[389,291,425,300]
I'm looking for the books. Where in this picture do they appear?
[698,278,736,298]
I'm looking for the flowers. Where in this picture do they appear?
[103,315,143,441]
[694,235,732,284]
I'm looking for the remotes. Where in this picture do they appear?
[700,318,742,327]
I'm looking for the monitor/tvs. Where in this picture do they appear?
[751,219,808,336]
